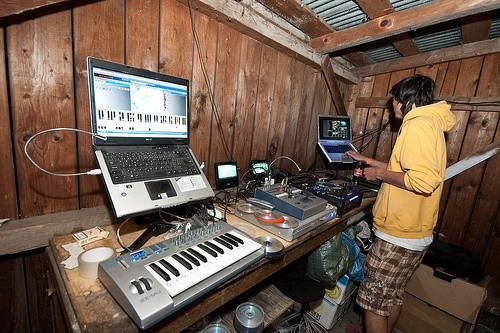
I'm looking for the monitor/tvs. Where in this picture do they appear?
[214,161,238,190]
[249,159,270,180]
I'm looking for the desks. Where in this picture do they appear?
[44,180,380,333]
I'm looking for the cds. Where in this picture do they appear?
[237,203,299,228]
[255,236,286,259]
[201,301,265,333]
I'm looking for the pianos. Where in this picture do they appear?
[96,219,267,332]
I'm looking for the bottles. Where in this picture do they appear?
[351,160,363,186]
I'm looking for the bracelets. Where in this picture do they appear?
[367,158,372,164]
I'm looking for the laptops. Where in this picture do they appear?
[316,114,361,163]
[85,55,215,218]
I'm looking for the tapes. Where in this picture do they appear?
[78,245,117,280]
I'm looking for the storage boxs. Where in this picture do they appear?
[393,263,493,333]
[302,293,354,330]
[325,273,360,307]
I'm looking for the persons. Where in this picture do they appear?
[345,74,456,333]
